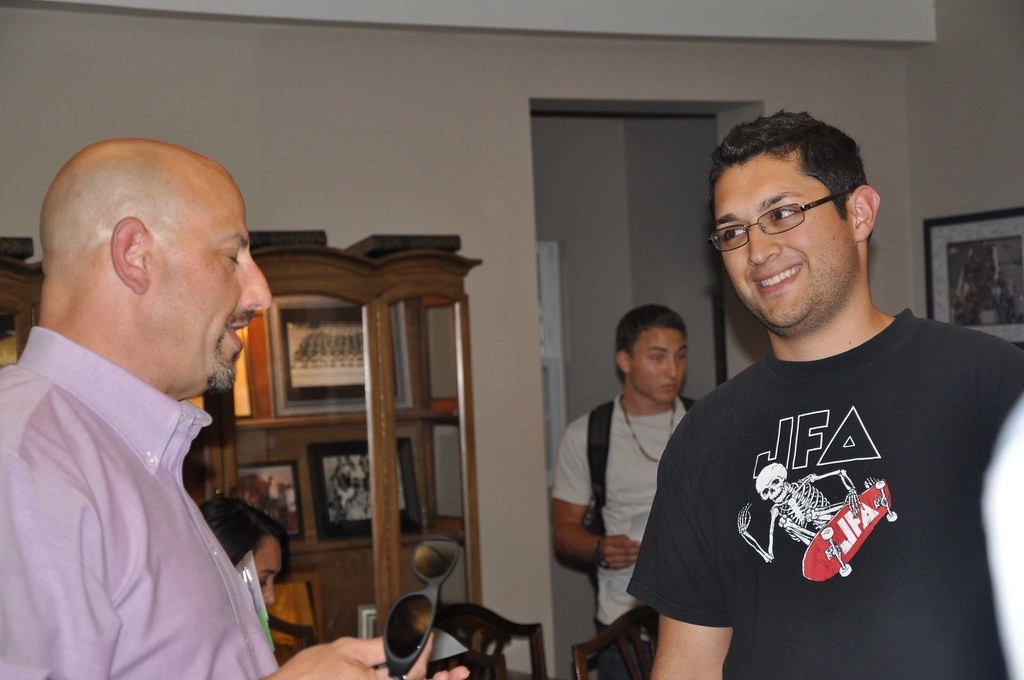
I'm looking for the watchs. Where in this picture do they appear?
[593,535,610,568]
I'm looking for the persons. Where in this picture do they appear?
[622,397,677,463]
[195,497,290,608]
[0,139,472,680]
[624,114,1024,680]
[552,303,697,680]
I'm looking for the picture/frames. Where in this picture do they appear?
[182,325,258,420]
[305,440,425,543]
[265,297,417,418]
[923,206,1024,349]
[236,460,305,541]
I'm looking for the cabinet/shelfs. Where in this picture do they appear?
[0,243,482,669]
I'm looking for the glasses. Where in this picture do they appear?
[710,188,854,251]
[382,537,460,680]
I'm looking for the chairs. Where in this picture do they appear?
[571,605,659,680]
[426,601,548,680]
